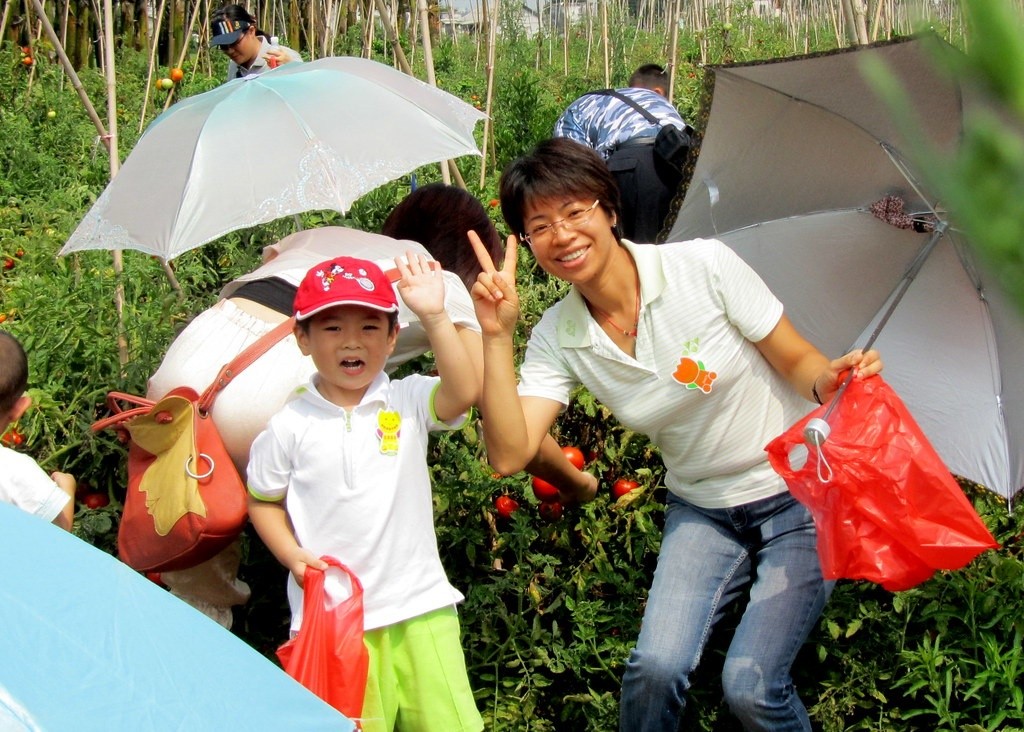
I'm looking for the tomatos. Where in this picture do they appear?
[22,47,183,118]
[144,572,163,586]
[0,249,110,508]
[471,95,482,110]
[495,446,641,521]
[490,199,498,207]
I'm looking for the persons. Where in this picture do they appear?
[468,138,883,732]
[210,5,305,80]
[245,251,487,732]
[553,87,692,243]
[0,329,77,532]
[146,181,598,641]
[626,64,668,98]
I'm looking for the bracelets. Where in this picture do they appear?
[811,376,824,405]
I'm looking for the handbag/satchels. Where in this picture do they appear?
[764,367,1000,591]
[653,124,693,192]
[90,385,249,574]
[275,556,369,732]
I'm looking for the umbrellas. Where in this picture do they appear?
[651,32,1024,520]
[58,56,495,268]
[0,500,357,732]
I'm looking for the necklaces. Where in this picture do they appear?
[593,271,640,337]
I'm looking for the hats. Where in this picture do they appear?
[210,18,251,45]
[293,257,399,328]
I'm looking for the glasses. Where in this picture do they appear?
[217,31,248,52]
[519,199,599,246]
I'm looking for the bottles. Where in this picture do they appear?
[266,36,280,55]
